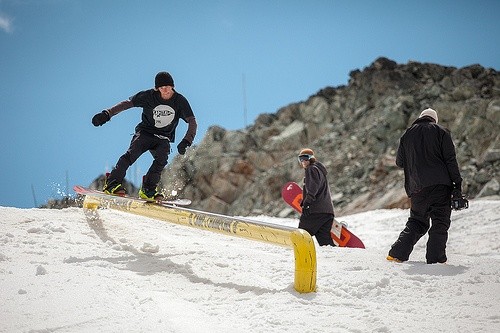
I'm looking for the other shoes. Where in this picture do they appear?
[387,255,403,263]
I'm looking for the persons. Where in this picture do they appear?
[386,109,463,264]
[298,148,335,247]
[93,71,197,200]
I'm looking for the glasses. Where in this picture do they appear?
[298,155,311,162]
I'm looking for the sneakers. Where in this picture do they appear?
[103,173,126,197]
[139,176,164,201]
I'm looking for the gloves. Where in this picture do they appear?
[452,178,463,198]
[177,139,191,155]
[92,110,110,126]
[302,201,311,216]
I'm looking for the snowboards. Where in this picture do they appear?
[73,185,192,206]
[282,183,365,249]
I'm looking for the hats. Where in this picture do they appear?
[419,108,438,124]
[300,149,315,161]
[155,71,175,89]
